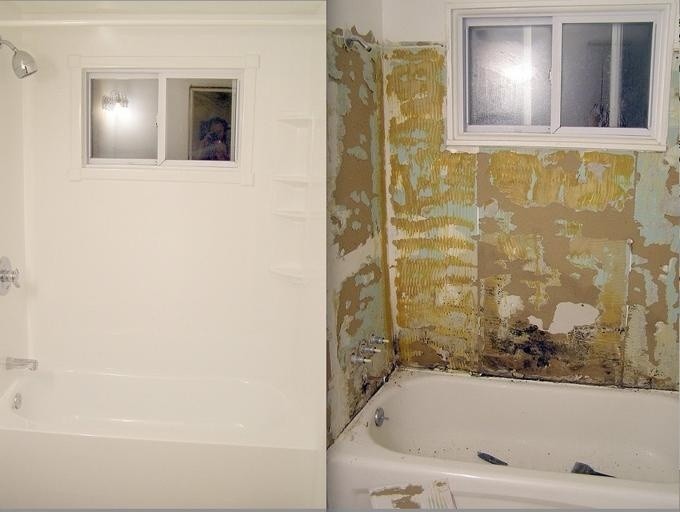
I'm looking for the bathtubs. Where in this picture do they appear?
[325,364,680,511]
[0,373,325,512]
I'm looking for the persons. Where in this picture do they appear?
[192,117,230,161]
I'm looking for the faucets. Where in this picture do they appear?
[6,356,40,374]
